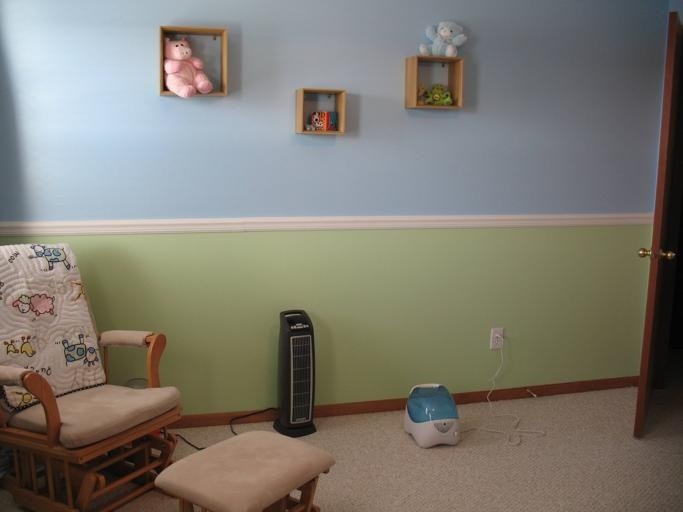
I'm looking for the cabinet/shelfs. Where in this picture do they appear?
[160,26,464,136]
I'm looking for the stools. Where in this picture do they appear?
[155,430,336,512]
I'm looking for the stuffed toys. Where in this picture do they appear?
[163,36,214,99]
[420,20,468,57]
[418,81,451,107]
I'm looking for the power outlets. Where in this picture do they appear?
[490,328,503,350]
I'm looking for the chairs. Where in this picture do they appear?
[0,242,182,511]
